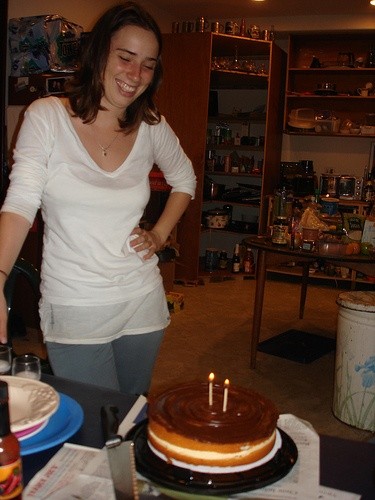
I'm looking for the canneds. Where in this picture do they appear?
[272,215,289,245]
[320,197,340,216]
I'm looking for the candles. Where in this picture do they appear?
[223,379,229,412]
[209,372,214,405]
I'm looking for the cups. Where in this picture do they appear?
[0,345,13,375]
[11,355,41,382]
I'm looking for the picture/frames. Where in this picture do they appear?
[320,174,337,198]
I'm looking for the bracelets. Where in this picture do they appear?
[0,270,8,278]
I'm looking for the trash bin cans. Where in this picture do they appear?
[336,290,375,435]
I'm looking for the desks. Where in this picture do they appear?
[241,236,375,366]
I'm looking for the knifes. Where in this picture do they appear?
[100,403,137,500]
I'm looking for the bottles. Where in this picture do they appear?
[0,380,23,500]
[171,14,374,279]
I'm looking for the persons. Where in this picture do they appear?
[0,4,196,397]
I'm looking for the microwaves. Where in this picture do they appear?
[319,174,363,199]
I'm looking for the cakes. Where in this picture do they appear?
[145,379,282,473]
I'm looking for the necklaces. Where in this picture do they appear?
[88,125,121,156]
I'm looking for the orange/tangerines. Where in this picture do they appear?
[344,242,361,255]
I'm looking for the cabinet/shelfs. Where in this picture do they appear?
[150,29,375,284]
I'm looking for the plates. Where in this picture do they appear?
[1,375,83,456]
[122,415,297,494]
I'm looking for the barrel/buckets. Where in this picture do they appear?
[332,291,375,433]
